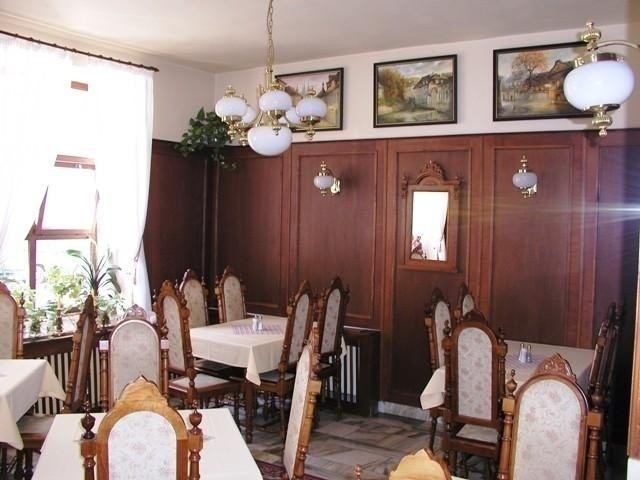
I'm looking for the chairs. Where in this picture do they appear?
[385,283,627,480]
[1,263,362,480]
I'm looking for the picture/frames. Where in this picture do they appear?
[372,54,458,129]
[490,39,594,120]
[273,66,344,133]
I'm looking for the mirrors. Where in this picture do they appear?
[398,158,464,276]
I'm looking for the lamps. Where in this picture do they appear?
[561,20,639,140]
[510,154,539,200]
[312,160,342,199]
[212,0,328,159]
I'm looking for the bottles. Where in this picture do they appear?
[251,313,263,332]
[517,343,532,362]
